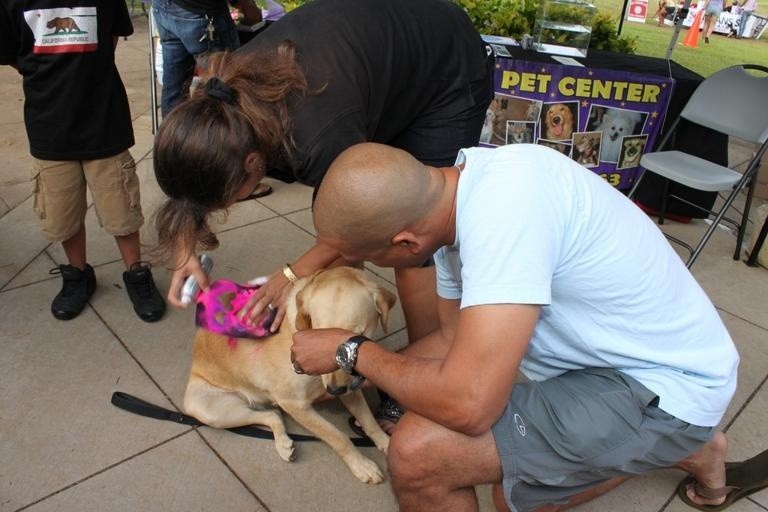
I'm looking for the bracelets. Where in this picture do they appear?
[283,263,299,284]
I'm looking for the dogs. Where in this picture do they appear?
[623,137,646,167]
[183,266,396,486]
[545,105,574,141]
[576,137,597,164]
[511,128,525,144]
[479,108,493,144]
[593,106,643,162]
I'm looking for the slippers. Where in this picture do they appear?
[678,449,768,512]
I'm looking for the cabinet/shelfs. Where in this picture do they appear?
[532,1,596,58]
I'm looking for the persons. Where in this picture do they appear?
[290,143,739,512]
[0,0,164,321]
[657,0,757,44]
[149,6,163,129]
[153,0,270,194]
[152,0,495,437]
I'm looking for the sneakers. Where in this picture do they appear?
[123,262,165,322]
[49,264,96,320]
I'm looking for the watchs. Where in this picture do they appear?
[335,335,371,374]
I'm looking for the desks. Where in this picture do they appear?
[657,5,768,38]
[481,39,728,221]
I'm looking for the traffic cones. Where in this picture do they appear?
[678,10,706,49]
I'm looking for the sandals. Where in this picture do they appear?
[234,183,272,201]
[348,396,405,440]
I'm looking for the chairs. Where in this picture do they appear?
[627,63,768,269]
